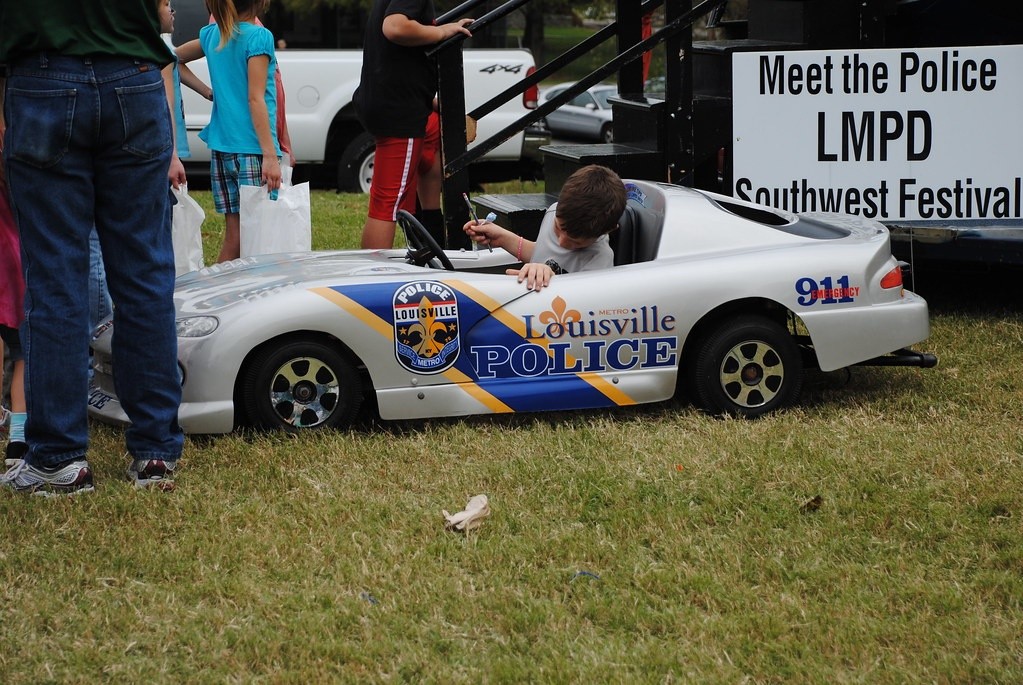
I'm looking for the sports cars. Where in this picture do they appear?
[85,178,939,448]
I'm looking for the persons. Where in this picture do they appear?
[0,0,296,498]
[463,165,627,292]
[352,0,475,249]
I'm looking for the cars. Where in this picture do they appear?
[643,75,667,100]
[537,80,665,143]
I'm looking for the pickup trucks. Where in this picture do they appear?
[155,1,537,197]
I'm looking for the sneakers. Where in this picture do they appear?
[0,459,94,499]
[127,457,177,491]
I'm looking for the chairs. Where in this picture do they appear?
[609,204,637,266]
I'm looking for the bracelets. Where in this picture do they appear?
[518,237,522,261]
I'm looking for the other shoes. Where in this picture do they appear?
[4,441,29,466]
[0,406,12,433]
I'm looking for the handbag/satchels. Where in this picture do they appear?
[240,182,312,265]
[169,182,207,278]
